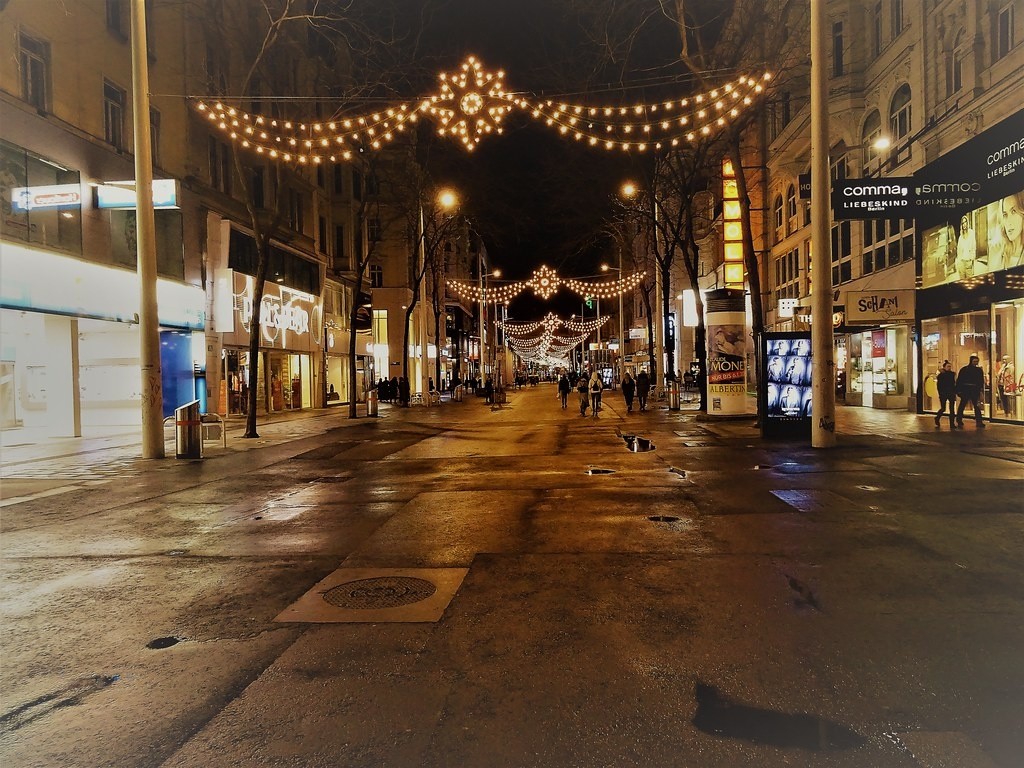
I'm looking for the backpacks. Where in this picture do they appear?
[579,382,586,393]
[592,379,600,391]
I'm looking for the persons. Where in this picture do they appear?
[955,355,986,427]
[377,365,605,417]
[933,359,958,428]
[637,370,650,411]
[677,369,696,391]
[996,354,1015,417]
[621,372,636,413]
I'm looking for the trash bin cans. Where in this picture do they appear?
[456,384,462,402]
[174,399,201,460]
[668,380,680,411]
[367,388,379,416]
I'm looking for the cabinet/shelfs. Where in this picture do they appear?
[850,368,898,395]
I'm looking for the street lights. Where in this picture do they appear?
[417,196,458,401]
[812,136,894,449]
[571,304,586,373]
[494,300,512,345]
[626,186,664,402]
[584,283,600,360]
[601,249,626,388]
[478,253,500,388]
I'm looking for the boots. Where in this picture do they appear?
[934,410,943,426]
[949,414,957,429]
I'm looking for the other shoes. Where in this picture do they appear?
[955,418,965,426]
[580,411,586,416]
[977,421,986,427]
[565,405,567,408]
[639,407,646,410]
[561,406,564,408]
[627,409,632,412]
[593,413,598,417]
